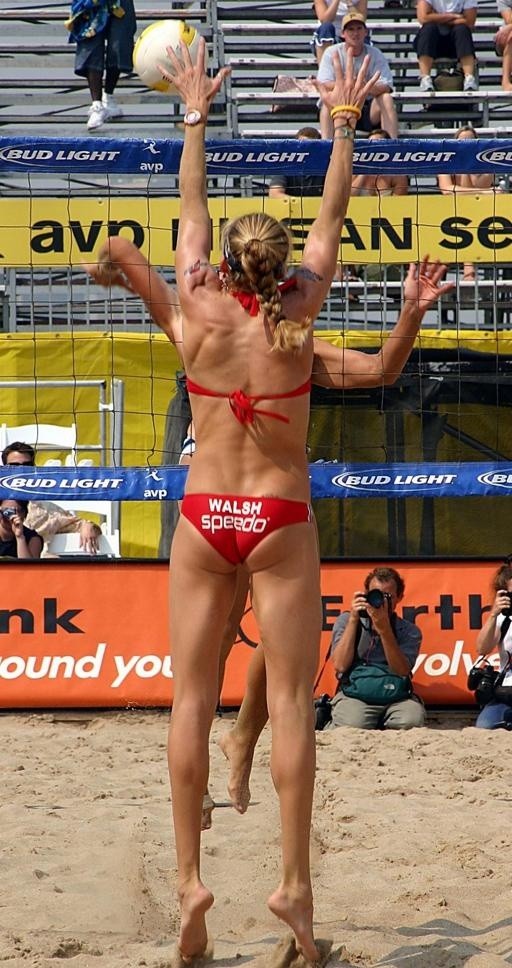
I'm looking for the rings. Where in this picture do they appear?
[91,541,95,544]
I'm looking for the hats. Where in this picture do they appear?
[341,12,366,31]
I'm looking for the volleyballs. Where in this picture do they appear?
[133,21,208,91]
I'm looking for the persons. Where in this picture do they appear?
[80,235,459,836]
[269,121,327,204]
[409,0,484,93]
[315,11,403,143]
[310,0,373,68]
[2,441,102,560]
[320,566,431,733]
[65,1,137,131]
[152,33,382,965]
[0,494,44,565]
[472,563,512,734]
[437,126,497,283]
[491,1,512,90]
[345,128,411,301]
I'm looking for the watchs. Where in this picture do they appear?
[179,107,205,129]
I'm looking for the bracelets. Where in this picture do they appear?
[329,105,364,119]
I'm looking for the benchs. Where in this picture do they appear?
[0,0,512,335]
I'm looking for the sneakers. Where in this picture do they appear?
[102,94,123,117]
[87,105,109,131]
[464,74,479,90]
[419,75,435,91]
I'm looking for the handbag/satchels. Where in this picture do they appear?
[340,661,413,705]
[271,75,320,112]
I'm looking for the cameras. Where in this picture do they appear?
[467,664,500,707]
[356,588,392,617]
[499,591,512,616]
[0,506,16,520]
[312,690,333,730]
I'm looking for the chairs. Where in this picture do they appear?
[37,499,124,562]
[1,419,79,468]
